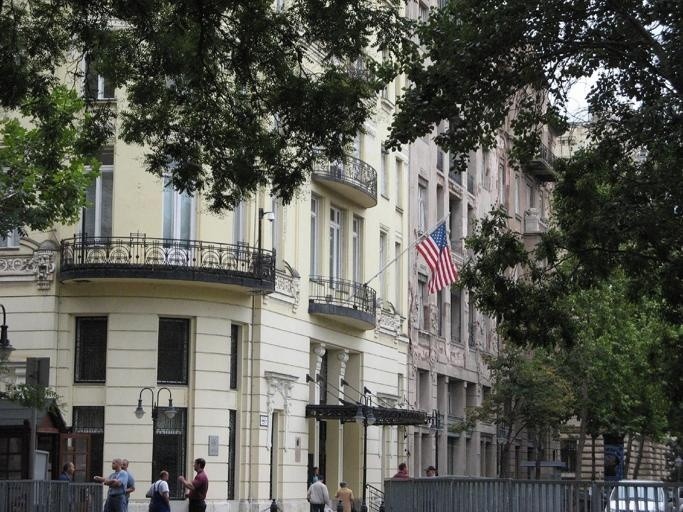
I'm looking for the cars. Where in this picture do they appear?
[604,480,683,512]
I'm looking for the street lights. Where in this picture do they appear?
[0,303,16,361]
[430,409,441,477]
[135,388,176,484]
[355,395,376,512]
[258,208,275,253]
[498,429,506,477]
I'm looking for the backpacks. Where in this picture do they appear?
[149,491,170,512]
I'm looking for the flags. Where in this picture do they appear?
[415,221,459,296]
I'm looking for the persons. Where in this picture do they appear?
[306,475,329,511]
[176,458,209,512]
[334,481,354,511]
[104,457,128,512]
[392,462,409,478]
[426,466,438,476]
[307,467,319,485]
[58,460,76,511]
[93,458,135,511]
[145,470,170,511]
